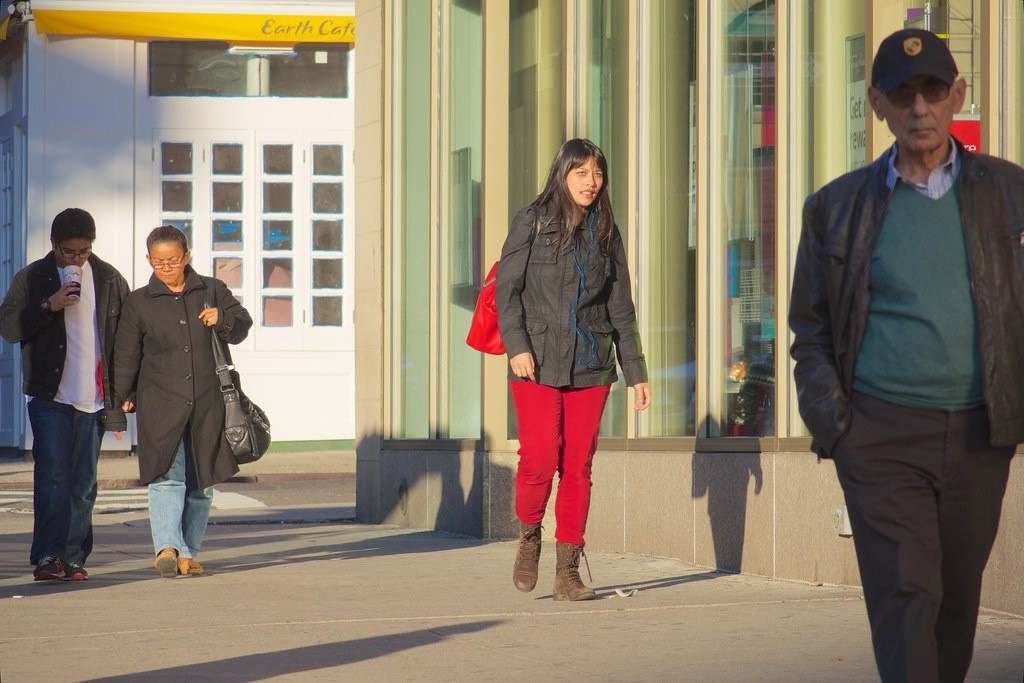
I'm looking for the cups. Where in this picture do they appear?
[62,265,83,304]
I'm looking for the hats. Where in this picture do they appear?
[868,29,961,92]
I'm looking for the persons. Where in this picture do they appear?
[0,209,135,581]
[111,225,254,578]
[789,28,1024,683]
[494,139,650,601]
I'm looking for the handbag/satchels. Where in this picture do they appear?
[466,205,540,357]
[219,383,271,466]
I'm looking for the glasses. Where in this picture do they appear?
[55,241,93,259]
[149,251,187,271]
[880,80,953,110]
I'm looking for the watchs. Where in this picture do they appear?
[39,298,51,313]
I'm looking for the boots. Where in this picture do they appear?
[550,541,597,603]
[512,520,544,593]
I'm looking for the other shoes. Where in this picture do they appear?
[175,558,205,575]
[33,555,66,582]
[58,560,90,581]
[153,550,181,579]
